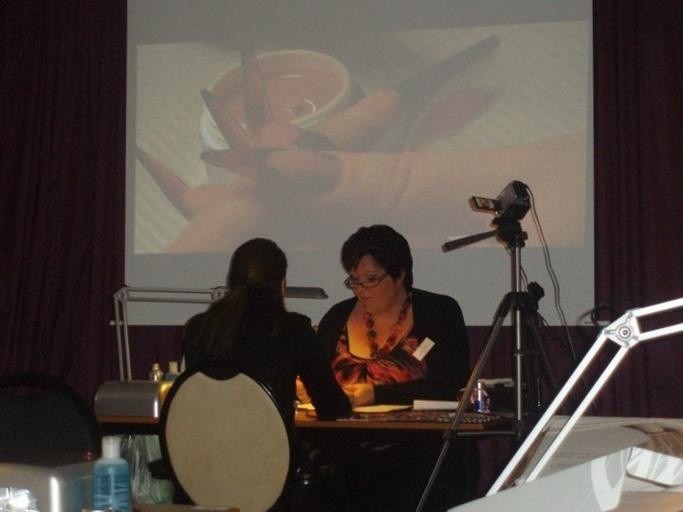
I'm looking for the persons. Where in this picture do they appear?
[295,224,471,406]
[177,236,355,511]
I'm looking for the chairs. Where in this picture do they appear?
[0,372,105,465]
[156,360,295,512]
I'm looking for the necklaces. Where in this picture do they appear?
[362,296,411,358]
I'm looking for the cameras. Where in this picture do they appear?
[468,180,531,228]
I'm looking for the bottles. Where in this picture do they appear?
[92,434,132,512]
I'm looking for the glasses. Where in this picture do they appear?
[343,273,389,289]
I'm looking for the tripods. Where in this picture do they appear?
[414,246,569,512]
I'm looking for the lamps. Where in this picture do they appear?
[95,285,328,418]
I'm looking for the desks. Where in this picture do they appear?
[448,412,683,512]
[95,408,533,433]
